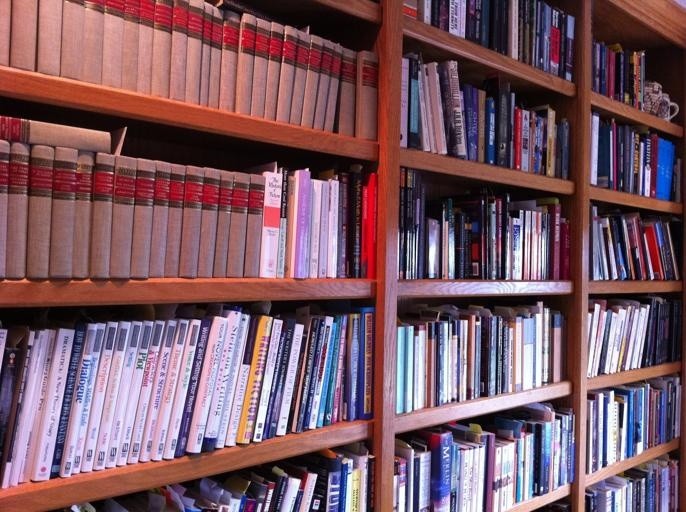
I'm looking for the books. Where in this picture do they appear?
[49,442,374,512]
[586,20,686,511]
[398,168,570,282]
[0,302,374,490]
[401,0,577,82]
[2,2,385,140]
[394,399,577,512]
[398,298,568,414]
[402,47,575,182]
[0,114,362,280]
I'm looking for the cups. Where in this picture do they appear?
[641,82,662,115]
[657,93,680,122]
